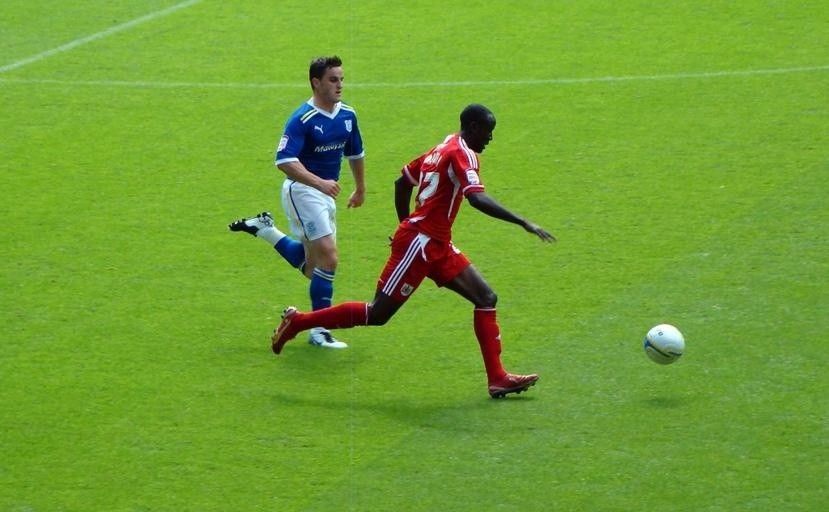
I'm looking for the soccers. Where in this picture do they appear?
[645,325,684,365]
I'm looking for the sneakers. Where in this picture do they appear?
[308,327,348,349]
[487,374,539,399]
[227,212,274,238]
[271,306,300,354]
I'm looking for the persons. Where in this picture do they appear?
[271,103,558,399]
[228,55,364,349]
